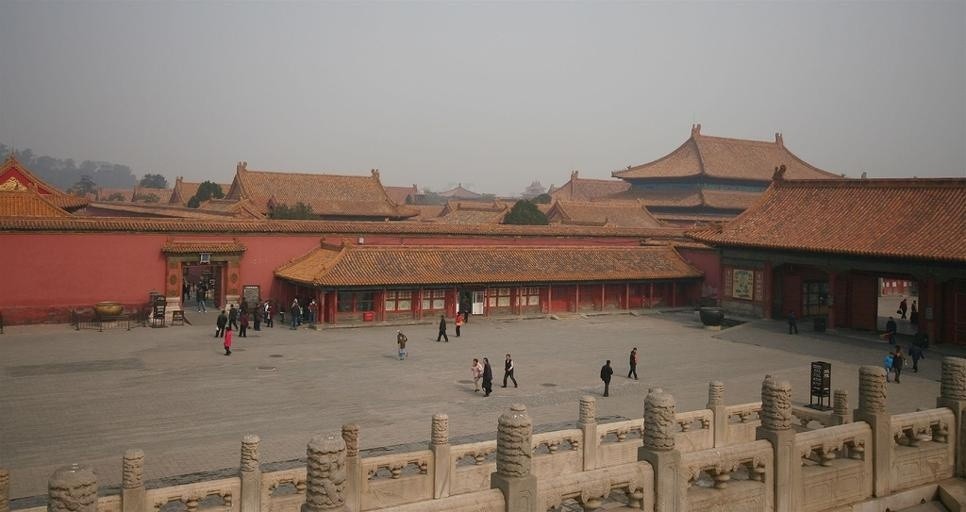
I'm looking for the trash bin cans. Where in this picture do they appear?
[814,317,825,332]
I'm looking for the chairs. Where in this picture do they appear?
[152,295,182,328]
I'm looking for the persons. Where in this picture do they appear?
[463,300,470,323]
[787,310,798,334]
[501,354,518,388]
[481,358,493,397]
[628,347,638,380]
[601,359,613,397]
[396,329,408,361]
[454,312,463,336]
[884,298,930,384]
[183,277,317,355]
[436,315,449,342]
[470,359,483,392]
